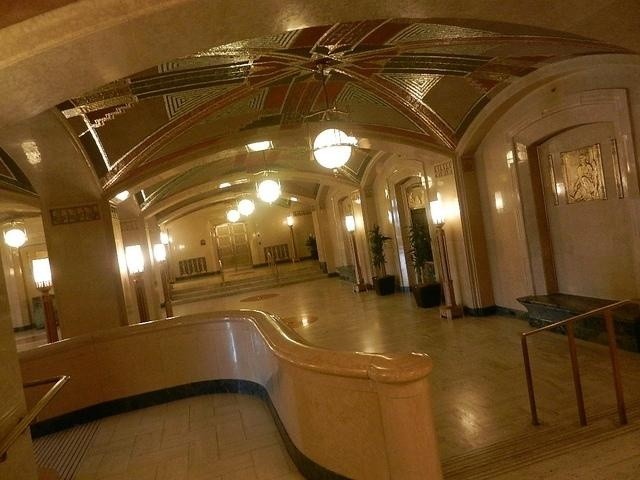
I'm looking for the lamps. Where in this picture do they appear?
[287,216,302,264]
[252,151,283,204]
[429,200,463,319]
[304,62,358,177]
[2,213,60,342]
[345,215,369,293]
[123,243,173,322]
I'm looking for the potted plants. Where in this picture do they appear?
[368,222,441,308]
